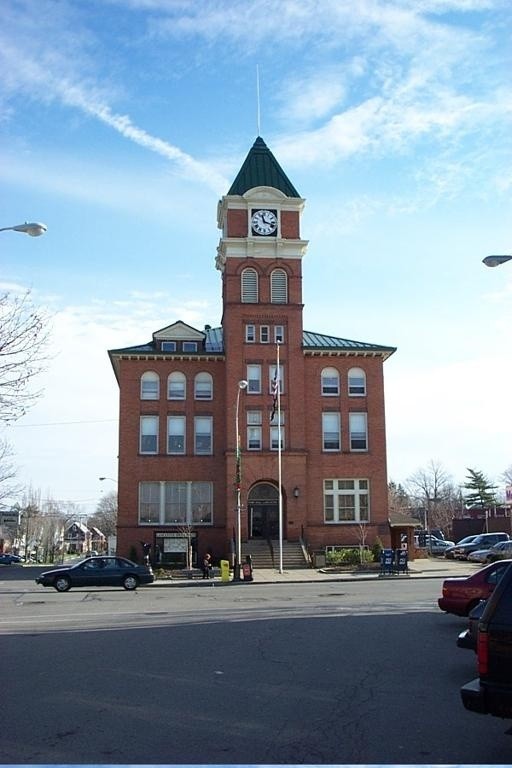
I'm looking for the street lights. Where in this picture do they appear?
[232,380,248,583]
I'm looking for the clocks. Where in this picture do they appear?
[251,208,278,236]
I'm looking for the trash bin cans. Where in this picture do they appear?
[379,548,408,570]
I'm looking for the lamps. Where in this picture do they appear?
[294,485,299,499]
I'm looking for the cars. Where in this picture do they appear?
[445,532,512,564]
[438,559,512,736]
[399,530,454,557]
[0,552,22,565]
[91,551,98,556]
[36,556,154,592]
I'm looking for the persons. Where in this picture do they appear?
[25,550,34,564]
[202,553,211,579]
